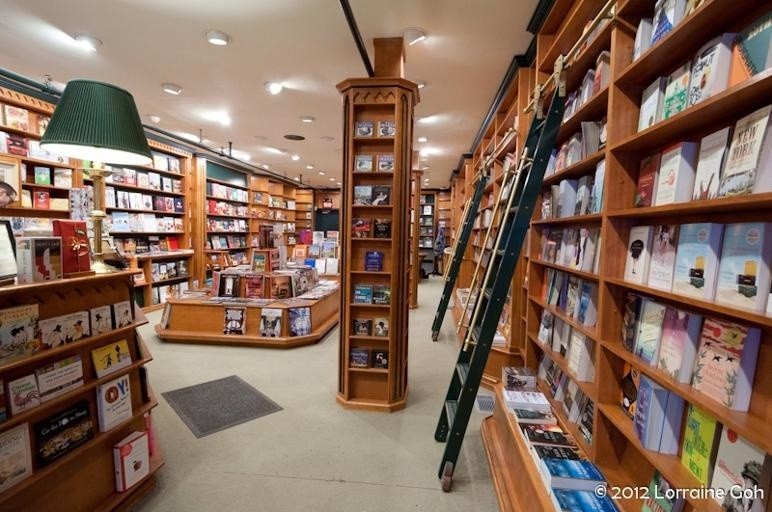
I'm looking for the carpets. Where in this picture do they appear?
[160,374,282,440]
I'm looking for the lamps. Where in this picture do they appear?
[38,81,154,274]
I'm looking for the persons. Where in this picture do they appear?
[0,180,19,210]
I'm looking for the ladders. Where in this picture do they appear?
[432,164,486,341]
[434,55,567,492]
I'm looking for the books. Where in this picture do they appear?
[111,236,180,254]
[121,168,135,186]
[506,391,549,407]
[349,348,368,369]
[707,426,766,511]
[149,150,180,172]
[142,195,152,211]
[686,34,733,104]
[136,173,147,188]
[632,19,649,57]
[647,228,677,291]
[171,179,180,192]
[696,317,764,412]
[129,192,141,212]
[456,287,506,348]
[28,217,49,235]
[532,443,582,460]
[539,267,596,327]
[112,166,121,182]
[552,489,616,512]
[0,424,31,495]
[30,400,94,467]
[623,227,652,284]
[287,309,310,336]
[640,472,686,511]
[208,199,246,216]
[617,362,639,418]
[219,274,239,298]
[21,189,30,206]
[368,184,390,207]
[438,221,445,229]
[522,428,572,447]
[420,195,433,249]
[118,191,127,210]
[1,305,38,362]
[53,198,71,211]
[374,218,390,240]
[637,78,662,129]
[8,132,28,156]
[354,284,372,302]
[163,198,173,212]
[128,214,185,232]
[92,340,131,380]
[378,121,393,135]
[631,377,686,455]
[152,197,162,210]
[677,405,718,484]
[354,121,372,136]
[295,230,340,300]
[562,52,611,118]
[244,274,263,298]
[716,103,772,197]
[7,375,39,414]
[206,183,248,201]
[33,192,51,208]
[536,310,596,385]
[512,409,557,426]
[113,430,151,492]
[540,161,605,217]
[152,261,189,279]
[103,164,111,182]
[112,299,131,329]
[208,235,246,251]
[19,235,63,281]
[36,310,89,351]
[149,173,160,190]
[251,252,264,274]
[353,318,370,337]
[353,155,371,172]
[364,252,382,272]
[538,228,599,268]
[209,253,246,267]
[260,308,282,338]
[664,64,692,117]
[374,285,390,303]
[34,164,49,186]
[655,141,695,204]
[98,375,133,434]
[152,282,190,306]
[376,154,394,172]
[53,167,72,189]
[537,357,596,436]
[373,319,389,337]
[551,119,609,166]
[4,216,25,235]
[632,154,659,206]
[208,217,246,231]
[174,199,183,212]
[620,293,647,352]
[104,186,114,208]
[269,275,293,300]
[6,105,28,130]
[671,225,720,301]
[501,365,537,388]
[351,217,372,238]
[223,307,245,336]
[33,354,83,402]
[717,224,770,311]
[89,306,110,338]
[352,185,372,206]
[370,349,388,369]
[37,117,50,136]
[651,0,687,39]
[653,307,705,388]
[54,220,89,275]
[729,11,770,87]
[162,177,170,193]
[693,128,730,200]
[535,457,607,488]
[112,212,127,231]
[633,301,666,364]
[250,192,295,249]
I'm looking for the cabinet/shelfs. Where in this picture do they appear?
[452,155,471,302]
[408,169,424,310]
[479,1,772,511]
[193,158,252,289]
[0,263,164,511]
[453,56,528,390]
[98,138,194,314]
[252,176,299,250]
[334,78,421,414]
[296,188,314,238]
[0,86,79,238]
[438,186,452,249]
[421,188,439,276]
